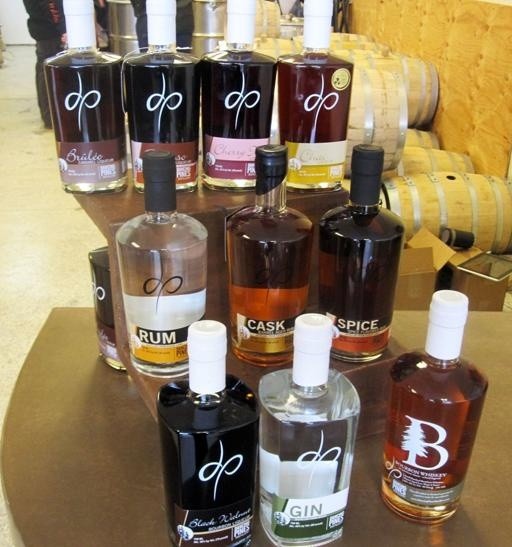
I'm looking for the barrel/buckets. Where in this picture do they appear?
[255,1,512,253]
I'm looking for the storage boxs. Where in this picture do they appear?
[392,225,485,310]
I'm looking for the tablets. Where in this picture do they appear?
[456,252,512,282]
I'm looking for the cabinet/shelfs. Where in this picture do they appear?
[1,161,512,547]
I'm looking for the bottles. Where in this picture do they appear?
[380,289,490,526]
[120,15,202,194]
[203,16,277,189]
[115,154,206,382]
[43,15,129,195]
[157,320,256,547]
[223,147,314,369]
[258,312,362,547]
[88,246,125,372]
[318,144,404,362]
[277,16,353,195]
[439,229,475,248]
